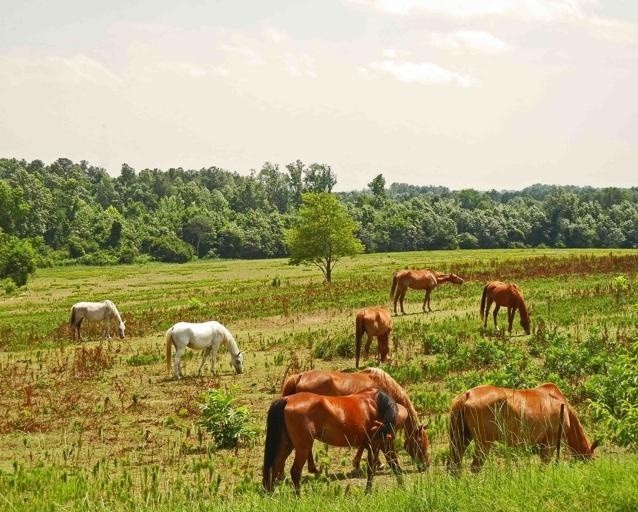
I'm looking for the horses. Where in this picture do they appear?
[69,300,127,342]
[390,268,463,316]
[263,387,408,498]
[355,306,393,369]
[165,321,246,380]
[276,367,430,481]
[446,382,605,478]
[481,280,531,335]
